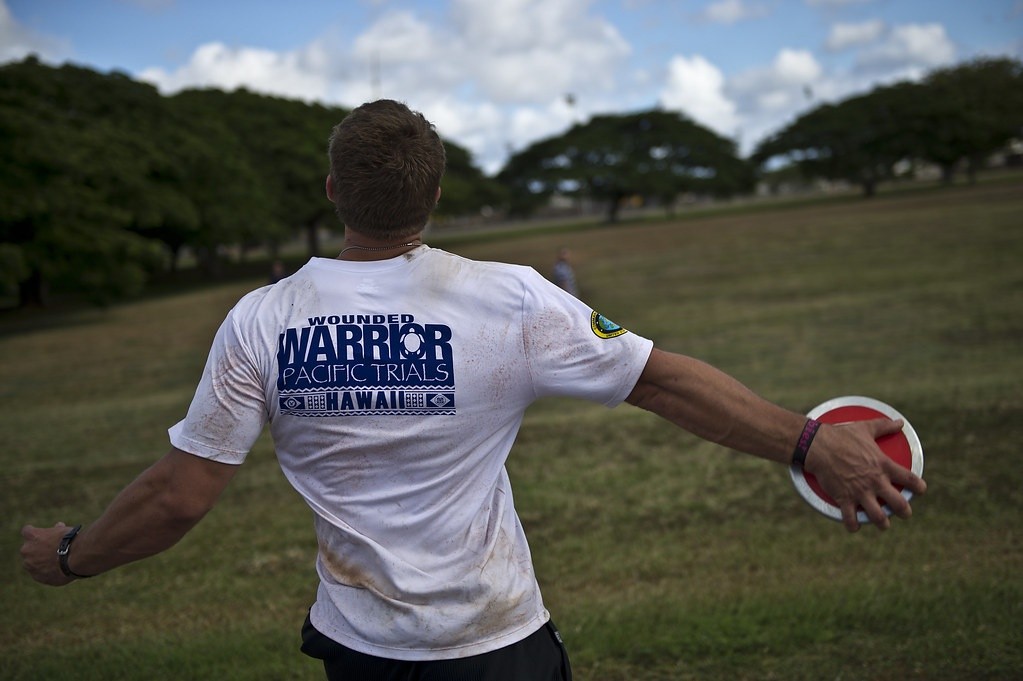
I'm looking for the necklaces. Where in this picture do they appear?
[339,242,421,257]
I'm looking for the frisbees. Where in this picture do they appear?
[791,394,925,526]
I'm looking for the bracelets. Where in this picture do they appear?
[792,418,822,468]
[56,525,93,579]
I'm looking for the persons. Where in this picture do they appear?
[19,100,928,681]
[552,245,575,294]
